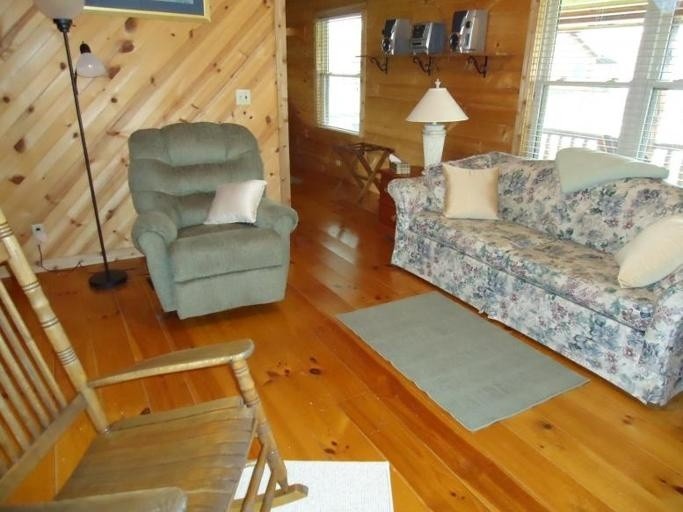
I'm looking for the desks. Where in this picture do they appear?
[377,164,424,228]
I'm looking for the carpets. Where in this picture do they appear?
[330,290,590,433]
[230,459,396,512]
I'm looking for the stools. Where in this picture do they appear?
[332,140,395,204]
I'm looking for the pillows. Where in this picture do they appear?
[438,145,681,290]
[201,179,268,227]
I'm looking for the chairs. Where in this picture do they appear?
[127,120,299,321]
[1,202,311,512]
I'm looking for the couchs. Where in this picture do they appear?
[385,149,682,408]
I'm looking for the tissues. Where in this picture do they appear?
[389,153,411,175]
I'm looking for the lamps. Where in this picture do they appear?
[30,0,131,291]
[404,78,470,175]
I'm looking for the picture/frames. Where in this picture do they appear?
[82,0,212,24]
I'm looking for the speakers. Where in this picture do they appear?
[381,19,410,55]
[449,9,489,54]
